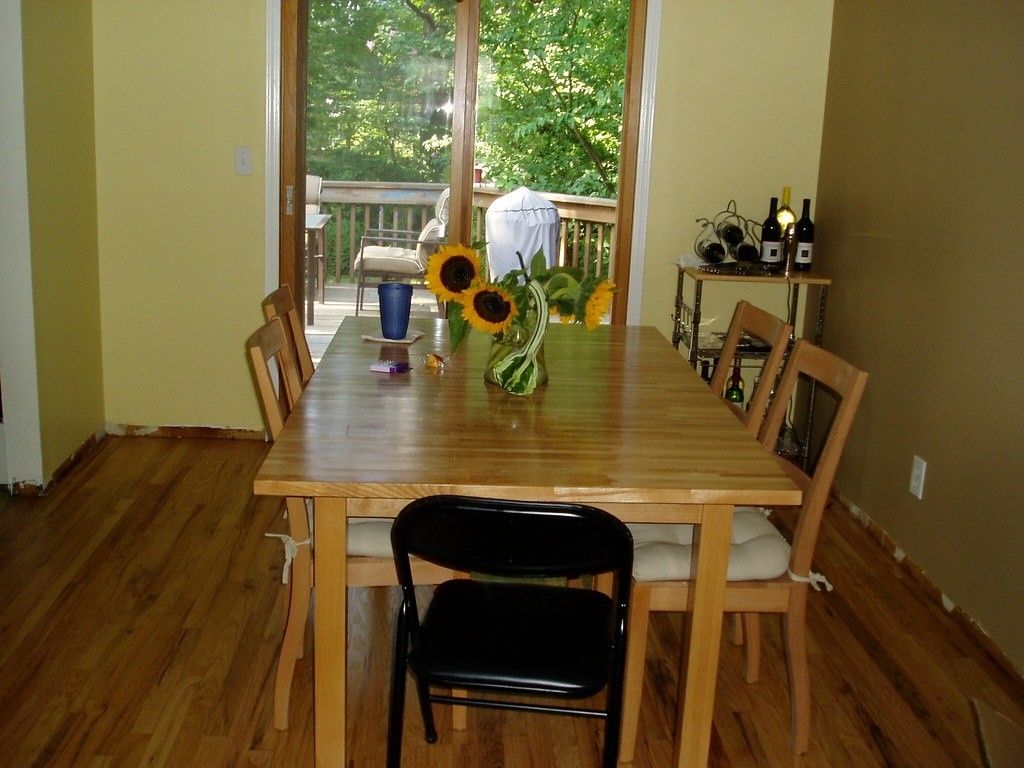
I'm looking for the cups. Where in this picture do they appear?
[377,282,414,339]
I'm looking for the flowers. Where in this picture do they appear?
[422,236,615,396]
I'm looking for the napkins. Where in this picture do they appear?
[360,327,427,344]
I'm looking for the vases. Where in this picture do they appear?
[483,309,549,388]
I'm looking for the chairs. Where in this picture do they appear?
[353,188,450,318]
[260,281,316,522]
[304,174,322,290]
[590,333,870,762]
[386,494,635,767]
[244,316,469,732]
[707,294,793,648]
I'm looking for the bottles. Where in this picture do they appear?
[697,219,760,266]
[758,197,782,274]
[776,186,815,273]
[701,357,785,424]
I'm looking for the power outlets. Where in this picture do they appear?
[908,454,926,499]
[235,146,252,175]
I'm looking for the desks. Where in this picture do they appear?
[305,215,332,325]
[253,314,803,768]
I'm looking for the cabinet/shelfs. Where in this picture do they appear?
[672,266,832,472]
